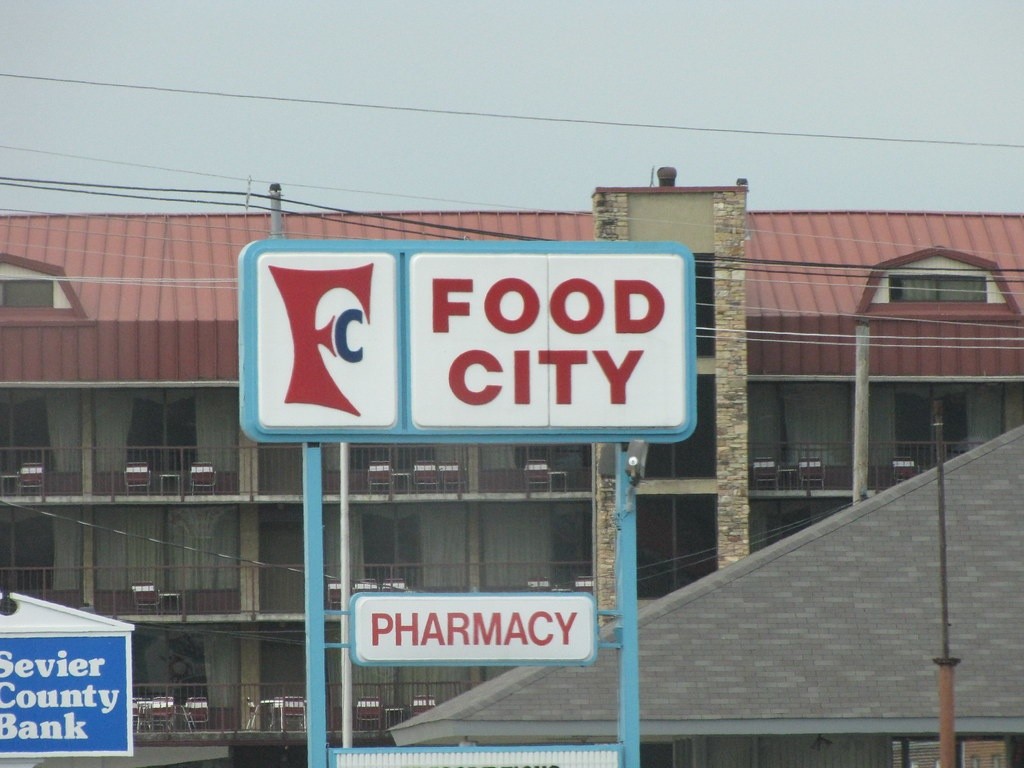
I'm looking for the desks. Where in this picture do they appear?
[546,471,569,492]
[0,474,21,496]
[549,587,573,593]
[384,707,405,730]
[775,467,798,491]
[158,473,182,496]
[158,592,182,616]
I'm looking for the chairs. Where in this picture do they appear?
[354,696,382,731]
[571,576,594,595]
[324,578,342,611]
[188,461,217,495]
[411,460,441,494]
[131,580,160,615]
[752,456,779,491]
[132,694,308,734]
[382,578,409,592]
[409,694,437,719]
[891,456,921,486]
[797,456,825,490]
[367,460,394,496]
[525,576,551,592]
[351,578,382,595]
[523,459,552,493]
[16,462,46,496]
[123,462,151,497]
[438,460,465,494]
